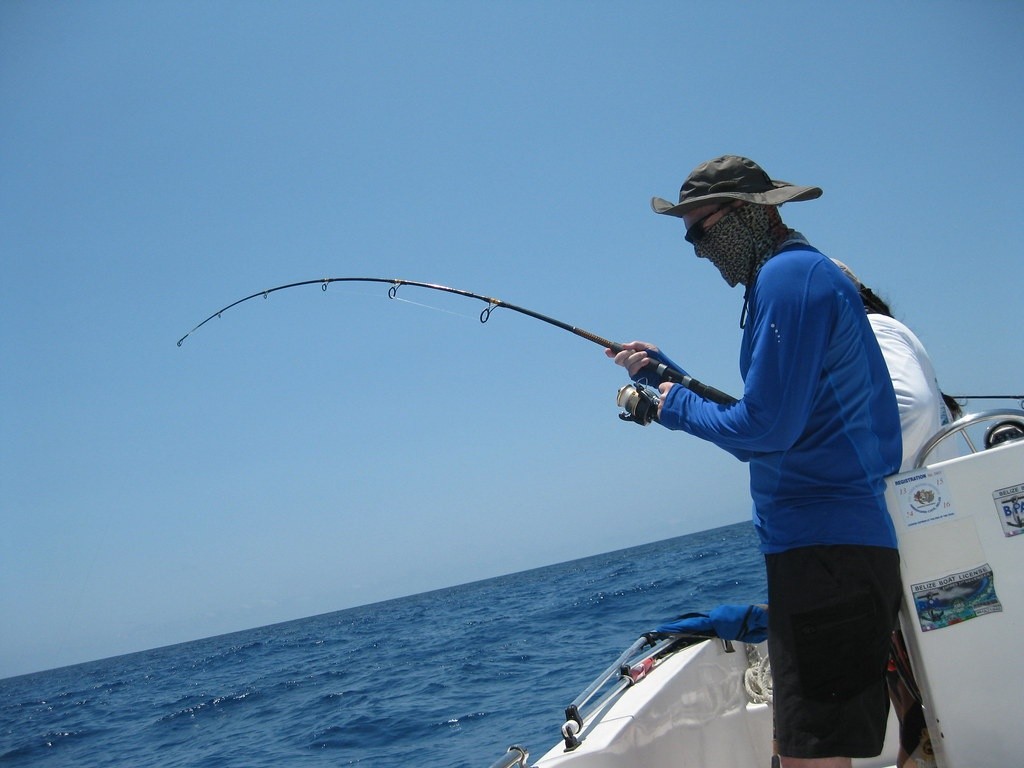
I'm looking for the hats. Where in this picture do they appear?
[651,154,824,218]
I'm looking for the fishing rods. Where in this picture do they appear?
[174,275,742,428]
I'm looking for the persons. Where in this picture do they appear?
[605,155,903,767]
[822,259,954,768]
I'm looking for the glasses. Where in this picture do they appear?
[684,200,739,244]
